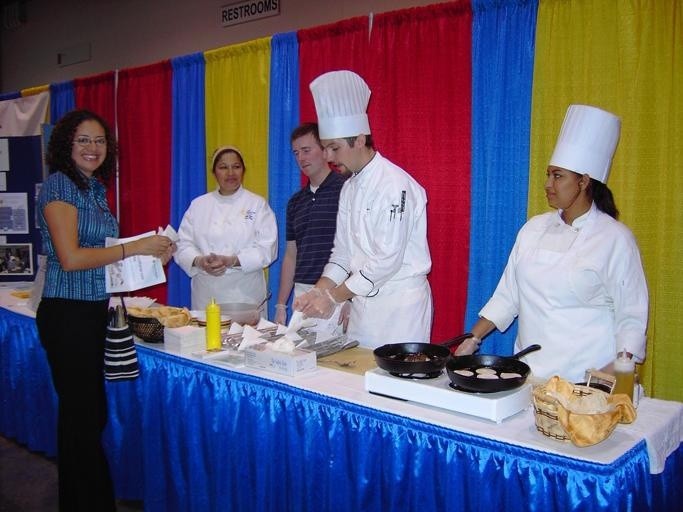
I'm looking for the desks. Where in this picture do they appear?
[0,256,683,512]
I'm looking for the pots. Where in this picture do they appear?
[445,344,543,394]
[372,332,475,377]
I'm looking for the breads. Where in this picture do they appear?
[10,291,31,298]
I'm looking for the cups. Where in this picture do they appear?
[613,348,636,417]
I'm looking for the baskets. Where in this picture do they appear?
[532,370,617,445]
[127,306,190,342]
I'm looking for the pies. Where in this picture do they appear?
[454,370,474,377]
[500,373,522,380]
[476,367,497,375]
[477,373,499,379]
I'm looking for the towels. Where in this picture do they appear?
[544,374,637,449]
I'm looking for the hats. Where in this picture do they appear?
[548,104,621,184]
[211,145,244,172]
[309,69,372,139]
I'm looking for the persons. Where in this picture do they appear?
[454,104,649,377]
[33,110,177,511]
[274,123,350,335]
[292,69,435,349]
[172,144,279,322]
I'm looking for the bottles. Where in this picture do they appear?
[204,295,223,350]
[113,305,126,328]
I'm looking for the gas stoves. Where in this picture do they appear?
[364,365,536,426]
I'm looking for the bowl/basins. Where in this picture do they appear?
[216,301,264,327]
[190,310,232,336]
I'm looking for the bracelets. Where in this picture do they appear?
[121,244,124,260]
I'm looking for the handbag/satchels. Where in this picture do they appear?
[103,321,140,382]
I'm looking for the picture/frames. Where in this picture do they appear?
[0,192,31,236]
[0,136,13,172]
[0,172,8,192]
[0,242,37,282]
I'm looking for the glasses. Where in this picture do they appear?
[73,136,109,148]
